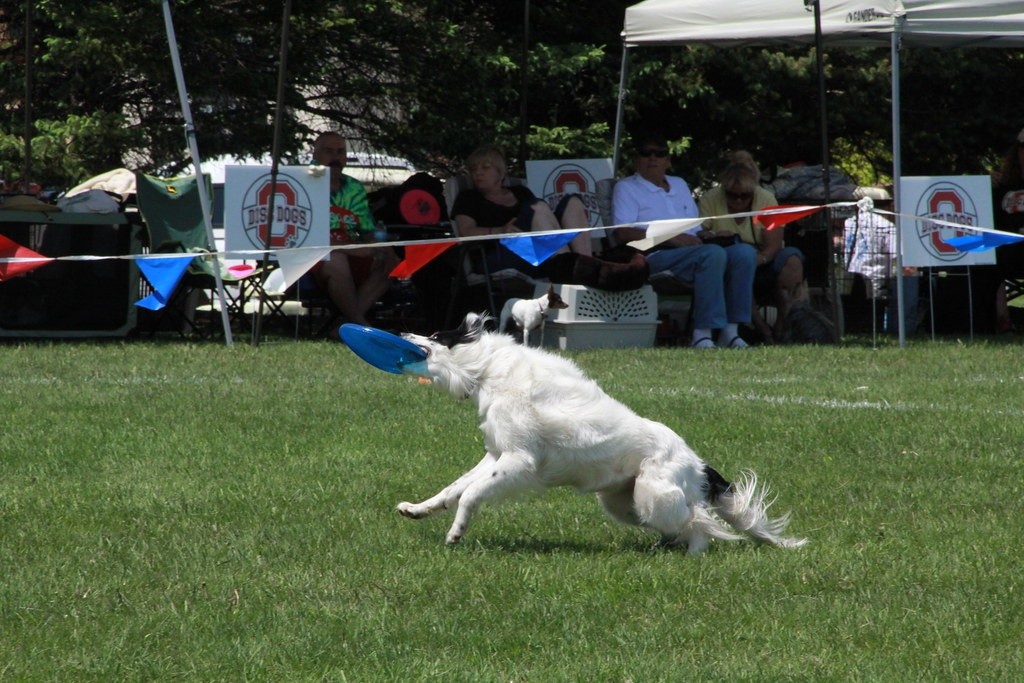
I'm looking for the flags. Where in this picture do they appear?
[389,242,456,280]
[752,205,821,230]
[499,232,581,267]
[263,250,331,296]
[943,232,1024,252]
[0,235,53,283]
[625,220,705,252]
[132,257,195,311]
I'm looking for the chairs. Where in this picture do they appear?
[775,194,836,346]
[381,172,451,335]
[443,171,534,328]
[134,169,288,348]
[593,184,698,344]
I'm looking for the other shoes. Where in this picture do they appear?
[691,338,716,348]
[728,336,750,349]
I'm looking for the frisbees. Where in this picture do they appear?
[338,320,426,377]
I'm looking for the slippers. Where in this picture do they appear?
[725,190,753,200]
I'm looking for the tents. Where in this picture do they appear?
[603,0,1024,345]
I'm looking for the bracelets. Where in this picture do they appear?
[759,253,767,265]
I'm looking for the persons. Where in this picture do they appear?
[610,134,757,348]
[448,144,592,279]
[989,126,1024,336]
[692,150,810,343]
[784,203,915,339]
[308,131,402,340]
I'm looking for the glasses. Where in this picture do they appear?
[638,149,671,157]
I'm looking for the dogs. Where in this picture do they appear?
[397,313,809,554]
[498,284,569,349]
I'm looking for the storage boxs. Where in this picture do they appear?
[533,283,660,351]
[0,210,140,337]
